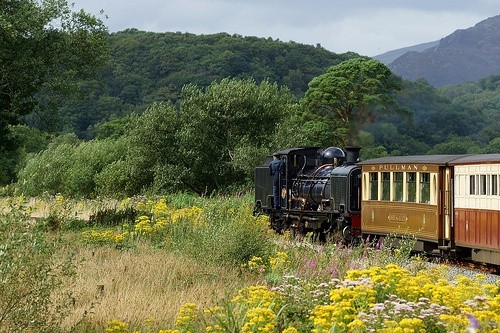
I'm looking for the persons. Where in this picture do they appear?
[268,153,286,210]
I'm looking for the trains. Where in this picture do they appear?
[253,146,500,271]
